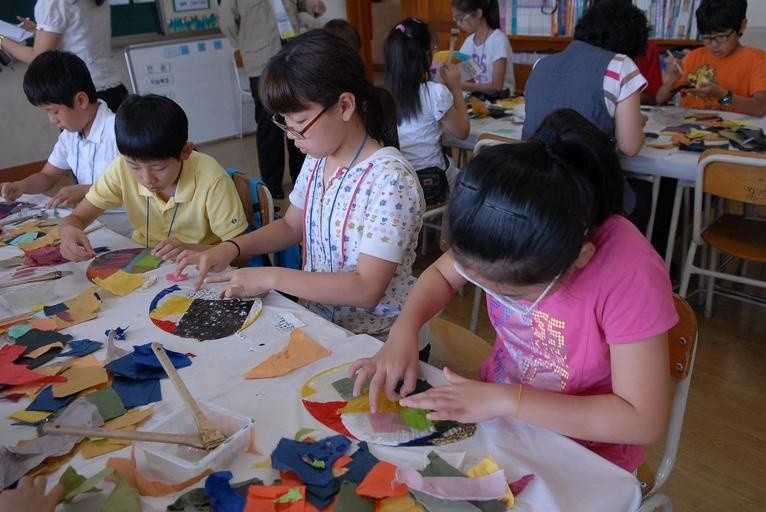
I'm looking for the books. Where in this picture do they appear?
[498,0,702,74]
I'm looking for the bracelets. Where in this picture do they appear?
[515,383,523,417]
[718,91,733,104]
[0,35,5,50]
[223,240,240,258]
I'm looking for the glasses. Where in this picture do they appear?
[453,229,591,316]
[700,28,735,46]
[451,12,474,25]
[270,98,337,141]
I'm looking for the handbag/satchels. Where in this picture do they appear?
[416,167,449,206]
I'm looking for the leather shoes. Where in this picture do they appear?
[269,186,285,199]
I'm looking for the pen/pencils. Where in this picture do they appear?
[18,17,30,27]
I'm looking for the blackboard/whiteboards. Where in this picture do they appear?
[123,35,277,149]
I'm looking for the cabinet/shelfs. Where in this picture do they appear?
[508,33,707,107]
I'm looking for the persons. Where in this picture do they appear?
[347,108,681,477]
[634,40,667,106]
[0,474,66,512]
[0,0,129,185]
[452,0,516,99]
[383,16,470,199]
[174,27,431,363]
[323,18,361,52]
[58,93,250,268]
[655,0,766,117]
[1,50,135,238]
[521,0,682,292]
[217,0,326,213]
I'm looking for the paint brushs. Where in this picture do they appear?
[151,341,226,447]
[665,48,685,75]
[447,28,460,66]
[0,270,73,289]
[42,423,204,450]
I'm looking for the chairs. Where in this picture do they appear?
[679,146,766,319]
[634,292,699,511]
[421,198,494,383]
[223,166,276,269]
[471,131,524,158]
[232,48,252,139]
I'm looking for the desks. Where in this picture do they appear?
[439,93,766,182]
[0,192,641,512]
